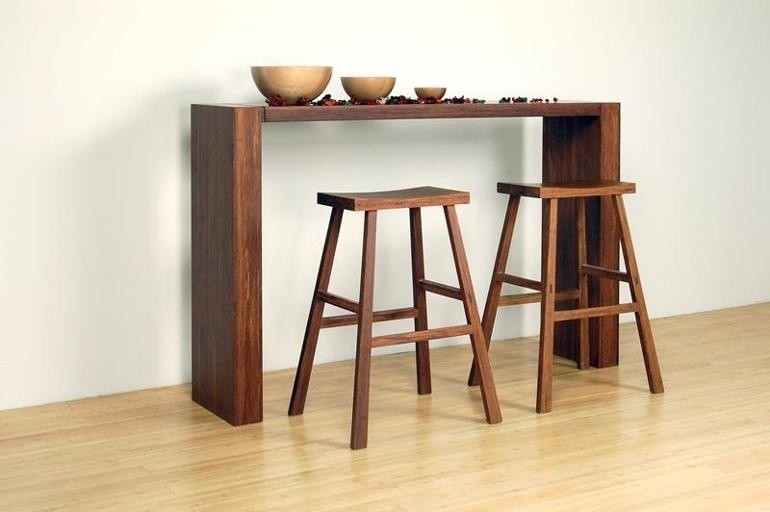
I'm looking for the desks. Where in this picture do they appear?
[192,102,621,426]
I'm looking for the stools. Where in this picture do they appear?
[288,187,503,450]
[469,183,664,414]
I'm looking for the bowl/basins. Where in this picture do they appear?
[339,77,397,105]
[413,87,447,104]
[250,66,333,105]
